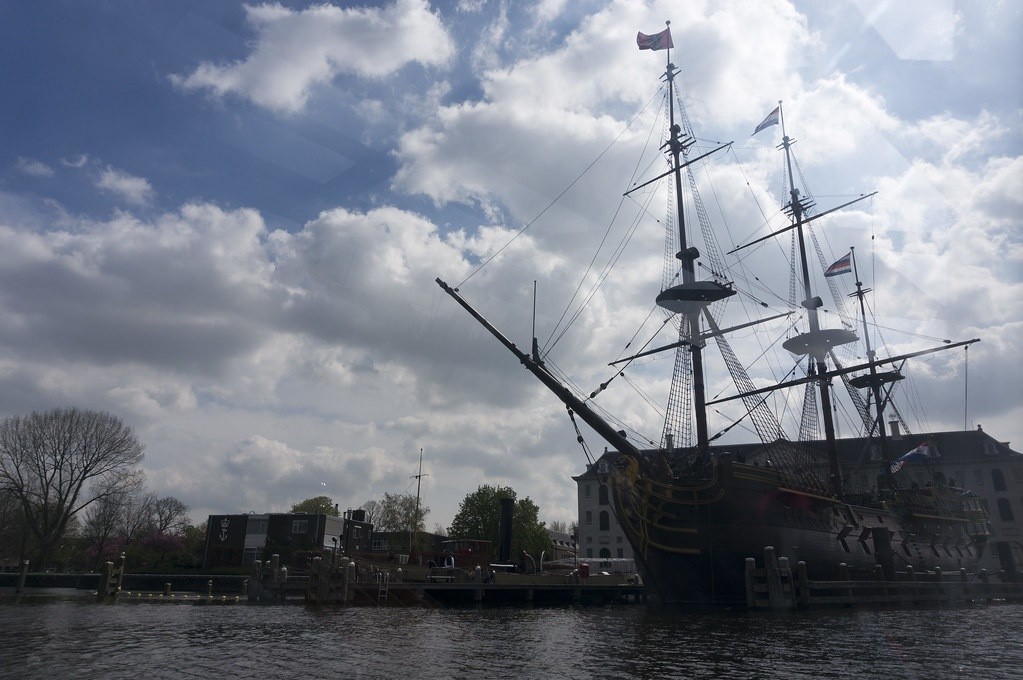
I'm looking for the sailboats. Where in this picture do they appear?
[437,20,993,619]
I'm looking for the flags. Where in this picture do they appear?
[890,441,930,474]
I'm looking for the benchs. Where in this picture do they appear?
[426,566,461,583]
